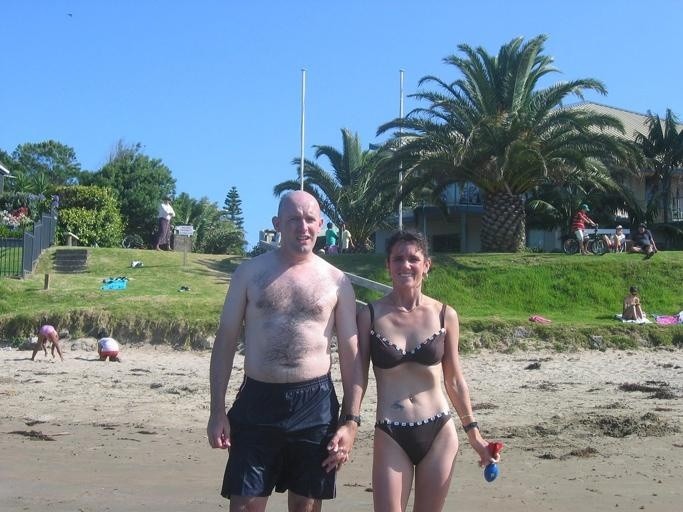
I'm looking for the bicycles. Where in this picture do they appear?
[562,223,610,256]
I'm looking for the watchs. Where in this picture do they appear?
[340,414,362,428]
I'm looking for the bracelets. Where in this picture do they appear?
[460,414,475,419]
[462,422,480,433]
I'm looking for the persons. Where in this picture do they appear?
[340,225,356,254]
[271,228,282,242]
[603,224,626,253]
[156,195,175,251]
[570,204,596,256]
[326,226,500,512]
[629,224,658,259]
[325,223,340,256]
[622,287,643,320]
[206,191,363,508]
[31,324,63,361]
[96,331,122,363]
